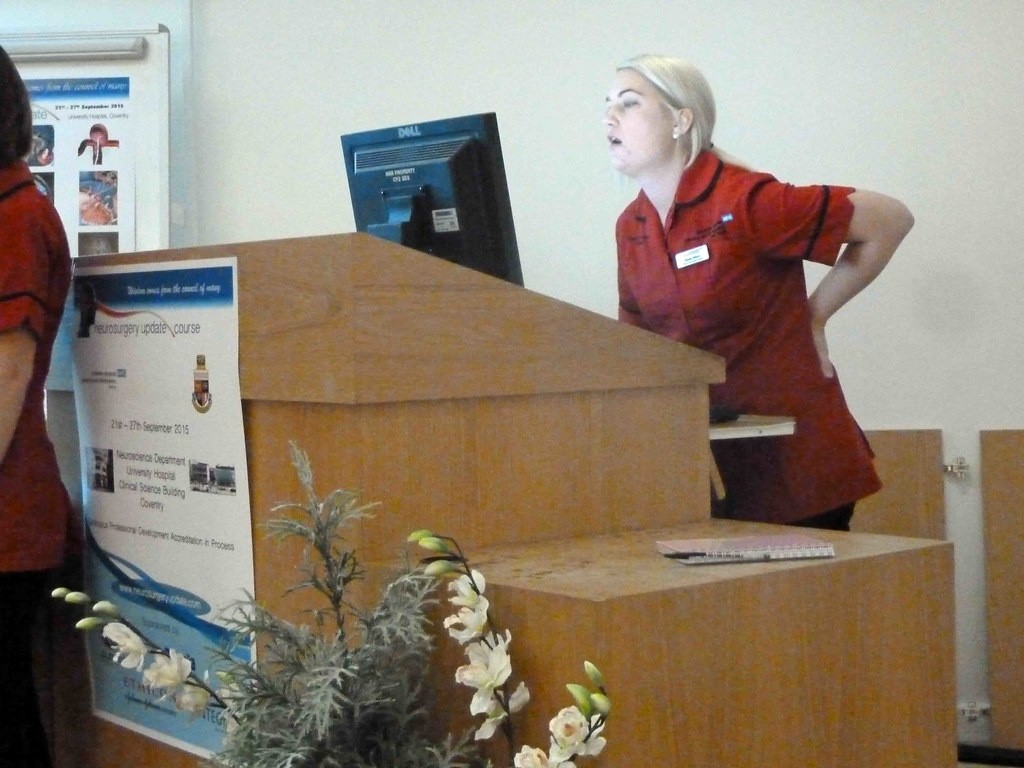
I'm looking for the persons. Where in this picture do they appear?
[0,42,73,768]
[602,50,914,533]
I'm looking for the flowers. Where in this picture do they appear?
[51,436,612,768]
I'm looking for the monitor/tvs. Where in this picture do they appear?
[340,112,525,287]
